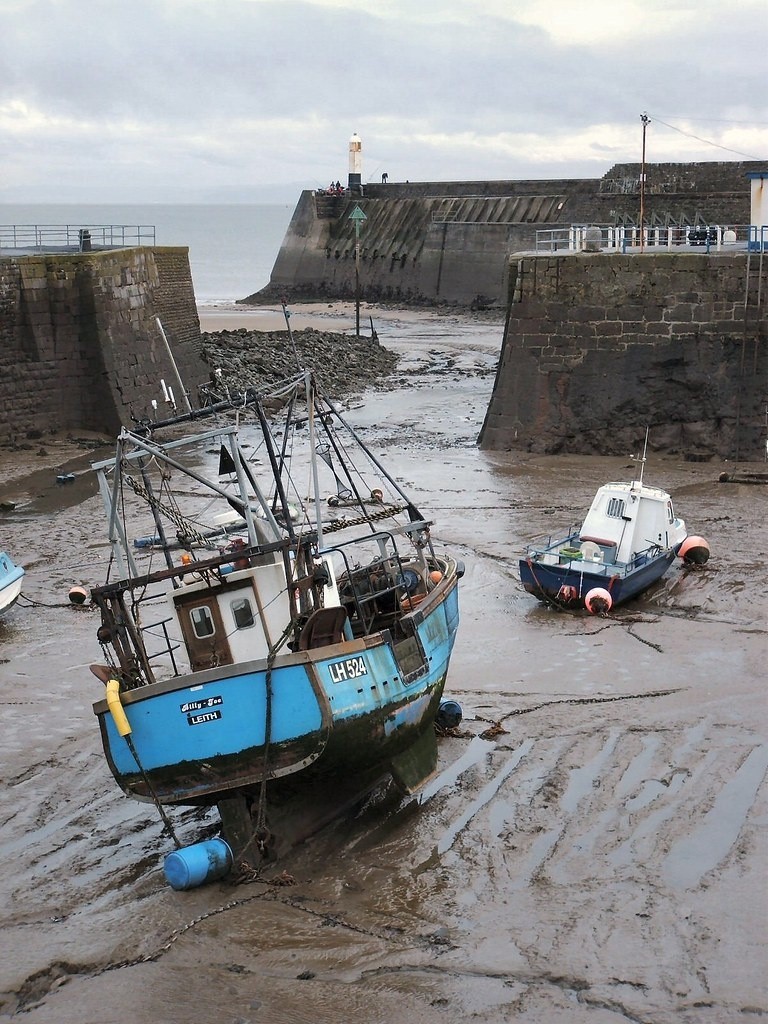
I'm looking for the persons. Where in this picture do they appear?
[209,363,222,390]
[381,173,388,184]
[328,181,344,195]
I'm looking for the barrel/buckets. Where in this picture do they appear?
[162,837,234,891]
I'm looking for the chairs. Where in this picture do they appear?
[580,541,604,565]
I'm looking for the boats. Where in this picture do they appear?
[0,553,26,615]
[91,312,465,893]
[518,427,711,619]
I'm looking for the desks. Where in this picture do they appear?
[564,560,607,575]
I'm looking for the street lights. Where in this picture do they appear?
[639,109,652,252]
